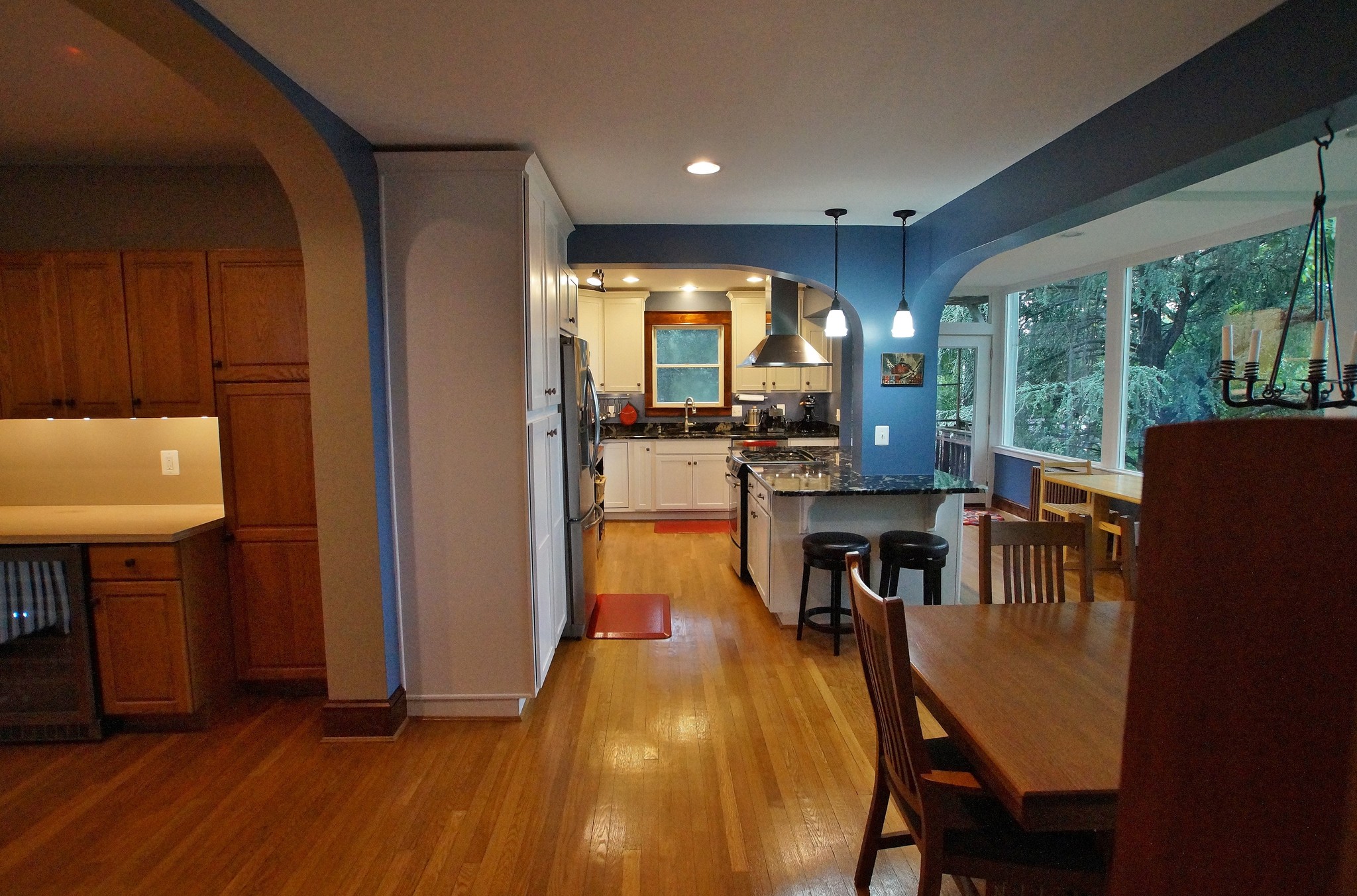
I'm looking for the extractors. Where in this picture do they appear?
[736,273,834,369]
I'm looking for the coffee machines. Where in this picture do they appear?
[762,405,784,434]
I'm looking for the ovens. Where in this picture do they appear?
[723,471,748,581]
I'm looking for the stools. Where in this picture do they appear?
[795,531,872,657]
[879,530,950,606]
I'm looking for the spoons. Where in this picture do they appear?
[618,403,619,415]
[620,403,621,414]
[607,402,610,417]
[613,404,616,414]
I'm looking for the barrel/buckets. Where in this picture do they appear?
[743,405,763,432]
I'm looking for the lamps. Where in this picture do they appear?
[1207,142,1357,411]
[586,269,607,293]
[891,209,915,339]
[823,207,848,338]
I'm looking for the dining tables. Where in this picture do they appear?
[897,599,1137,842]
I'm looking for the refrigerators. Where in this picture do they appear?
[561,333,606,636]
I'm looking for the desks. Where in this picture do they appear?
[1046,473,1143,570]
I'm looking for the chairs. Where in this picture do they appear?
[844,550,1097,896]
[977,514,1095,602]
[1038,459,1121,565]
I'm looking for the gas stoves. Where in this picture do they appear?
[725,446,825,476]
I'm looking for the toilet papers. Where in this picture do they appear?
[738,394,765,402]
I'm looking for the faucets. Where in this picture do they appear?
[684,397,697,431]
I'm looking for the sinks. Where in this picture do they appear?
[663,430,725,437]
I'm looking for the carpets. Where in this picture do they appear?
[654,520,730,534]
[586,594,672,640]
[963,508,1004,526]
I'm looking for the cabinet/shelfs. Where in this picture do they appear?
[656,439,730,512]
[592,439,634,512]
[122,247,216,422]
[206,243,330,702]
[368,150,579,720]
[801,316,832,393]
[745,472,965,629]
[87,525,236,733]
[633,439,652,513]
[726,289,804,394]
[0,250,133,418]
[578,288,650,394]
